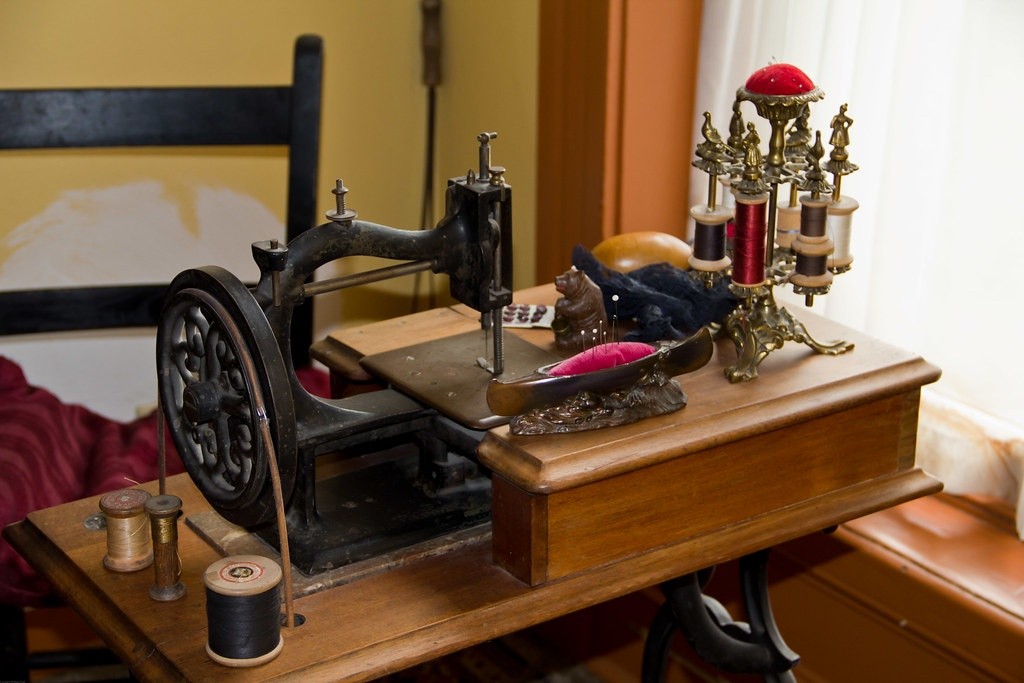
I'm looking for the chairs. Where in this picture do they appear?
[0,27,330,683]
